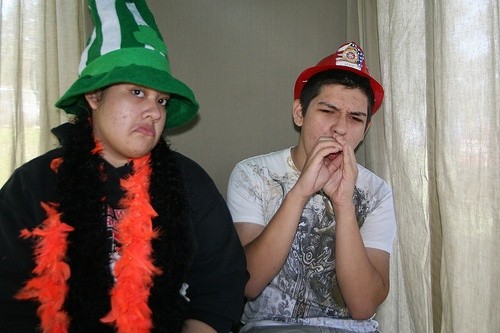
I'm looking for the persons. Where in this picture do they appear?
[224,40,399,333]
[0,0,251,333]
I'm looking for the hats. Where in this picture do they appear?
[293,42,385,116]
[53,0,199,129]
